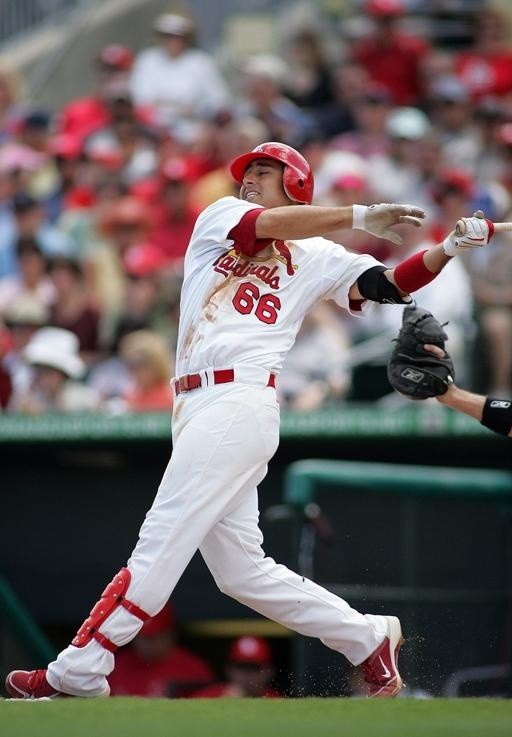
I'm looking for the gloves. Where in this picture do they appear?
[443,210,495,256]
[352,203,426,245]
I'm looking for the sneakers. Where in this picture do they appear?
[361,615,403,698]
[5,669,111,699]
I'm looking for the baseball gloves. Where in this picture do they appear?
[389,304,458,399]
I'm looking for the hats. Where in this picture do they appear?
[230,142,314,205]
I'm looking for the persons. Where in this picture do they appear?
[6,141,494,700]
[106,600,284,698]
[424,344,512,438]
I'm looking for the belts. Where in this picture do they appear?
[175,369,275,395]
